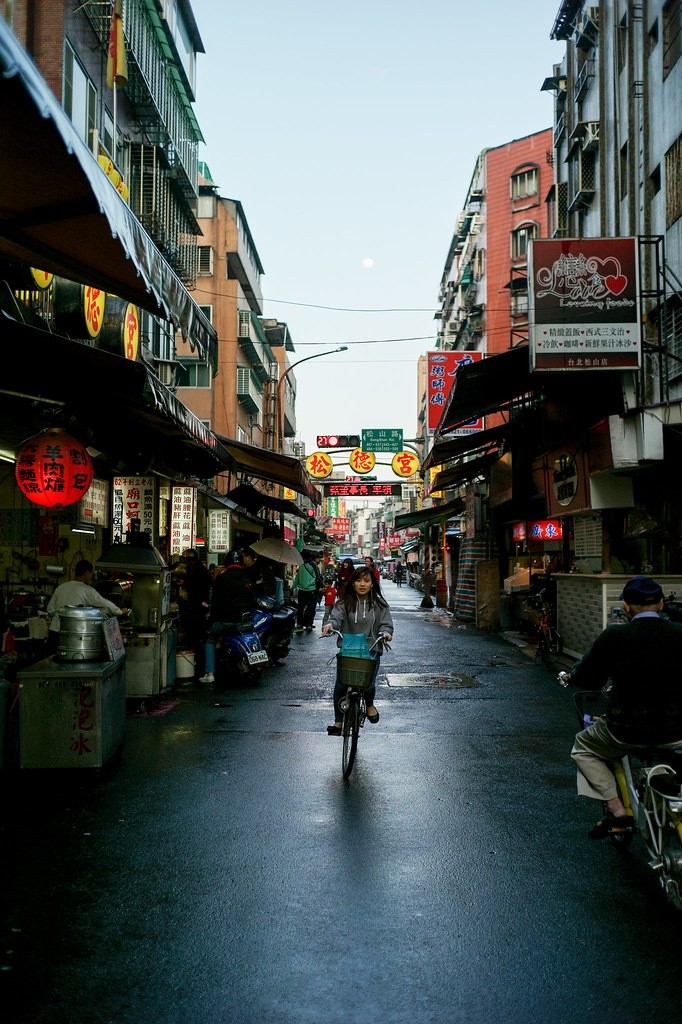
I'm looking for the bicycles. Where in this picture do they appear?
[523,587,563,664]
[319,626,392,778]
[558,672,682,916]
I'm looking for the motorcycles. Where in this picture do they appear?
[202,589,299,689]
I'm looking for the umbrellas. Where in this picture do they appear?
[249,537,304,568]
[107,0,128,162]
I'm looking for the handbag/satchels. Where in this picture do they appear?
[342,632,373,685]
[315,578,324,591]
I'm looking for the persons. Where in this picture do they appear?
[393,561,404,587]
[557,576,682,833]
[291,551,324,632]
[240,549,260,600]
[0,638,46,689]
[46,560,128,657]
[322,579,338,626]
[365,557,380,585]
[198,550,254,683]
[179,549,210,675]
[334,559,355,601]
[322,567,394,736]
[573,525,627,573]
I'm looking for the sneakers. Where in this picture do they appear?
[199,672,215,683]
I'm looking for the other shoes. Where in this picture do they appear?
[307,625,311,631]
[327,724,342,733]
[294,626,303,632]
[367,706,379,723]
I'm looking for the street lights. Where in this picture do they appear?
[278,346,348,579]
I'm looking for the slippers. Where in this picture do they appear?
[588,810,634,840]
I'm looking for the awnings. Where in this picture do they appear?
[212,432,321,507]
[0,27,237,478]
[224,484,308,520]
[395,497,466,531]
[420,422,510,480]
[434,344,547,437]
[430,451,499,494]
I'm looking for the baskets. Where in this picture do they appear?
[573,690,608,730]
[336,652,378,688]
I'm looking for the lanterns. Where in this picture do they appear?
[15,428,92,511]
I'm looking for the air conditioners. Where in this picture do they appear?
[447,321,461,332]
[558,80,566,93]
[583,121,598,150]
[581,5,598,27]
[576,23,582,43]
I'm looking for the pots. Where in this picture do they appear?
[57,603,106,660]
[6,587,51,617]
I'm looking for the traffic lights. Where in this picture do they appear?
[305,509,316,533]
[317,435,360,448]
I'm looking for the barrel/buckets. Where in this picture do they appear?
[175,650,196,678]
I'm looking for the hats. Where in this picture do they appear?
[242,548,255,560]
[619,575,663,606]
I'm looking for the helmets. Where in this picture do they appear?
[224,551,244,568]
[182,549,198,560]
